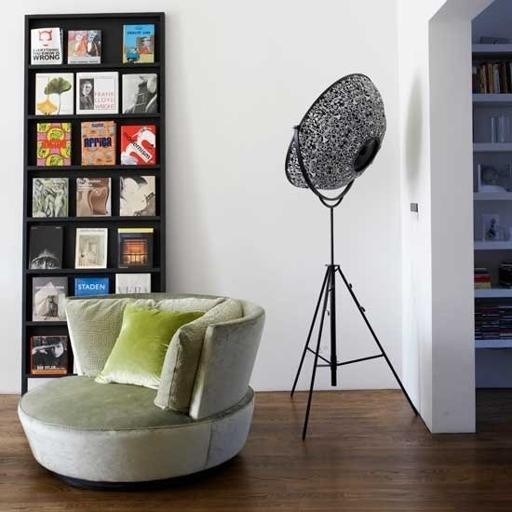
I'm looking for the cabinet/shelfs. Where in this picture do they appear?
[24,12,165,395]
[471,43,512,348]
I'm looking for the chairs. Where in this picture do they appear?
[17,293,266,490]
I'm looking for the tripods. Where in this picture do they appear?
[289,263,419,442]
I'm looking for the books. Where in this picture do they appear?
[472,61,512,94]
[32,277,68,322]
[74,273,151,296]
[474,268,491,289]
[29,23,160,270]
[30,336,67,374]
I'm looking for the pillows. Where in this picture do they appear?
[153,299,242,414]
[95,302,203,390]
[62,298,222,376]
